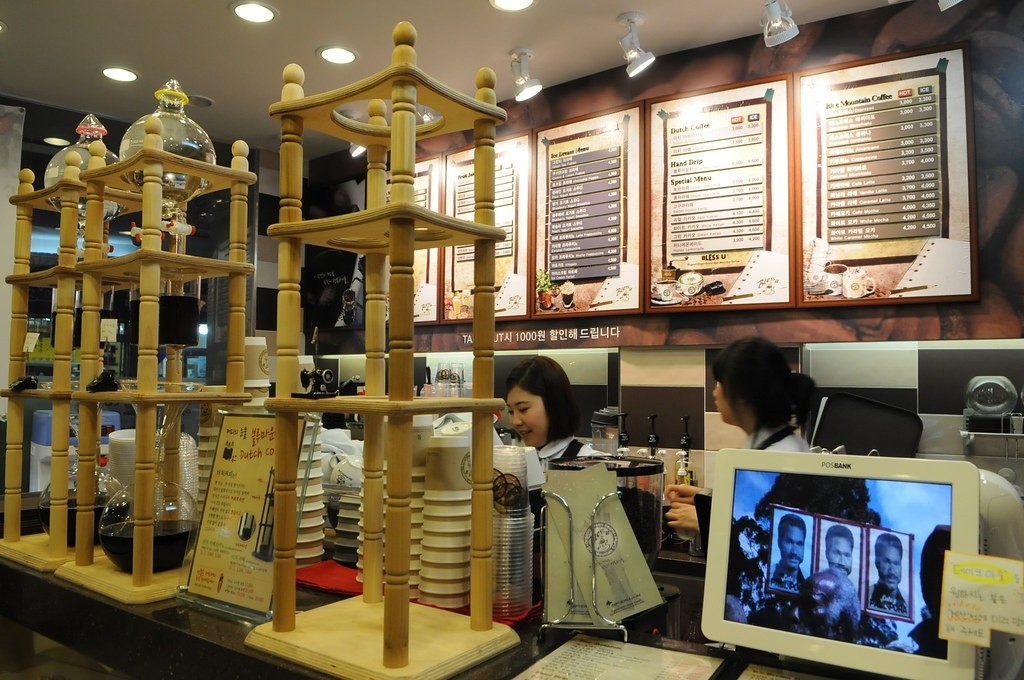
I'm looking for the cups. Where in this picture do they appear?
[329,415,531,615]
[561,292,573,307]
[432,363,473,398]
[678,273,703,294]
[197,336,327,567]
[823,264,848,296]
[843,274,876,298]
[651,279,678,300]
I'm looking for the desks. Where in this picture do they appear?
[0,555,847,680]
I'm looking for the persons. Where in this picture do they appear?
[664,336,815,532]
[769,513,806,595]
[789,567,860,643]
[869,533,908,617]
[825,524,854,576]
[505,356,612,461]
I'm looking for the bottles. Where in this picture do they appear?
[38,380,130,547]
[99,379,201,572]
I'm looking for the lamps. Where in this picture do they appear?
[758,0,799,48]
[615,10,656,78]
[349,142,366,157]
[508,46,543,102]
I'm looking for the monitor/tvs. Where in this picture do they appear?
[701,448,1024,680]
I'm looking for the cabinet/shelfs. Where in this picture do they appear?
[0,21,524,680]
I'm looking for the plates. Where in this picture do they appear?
[651,298,682,304]
